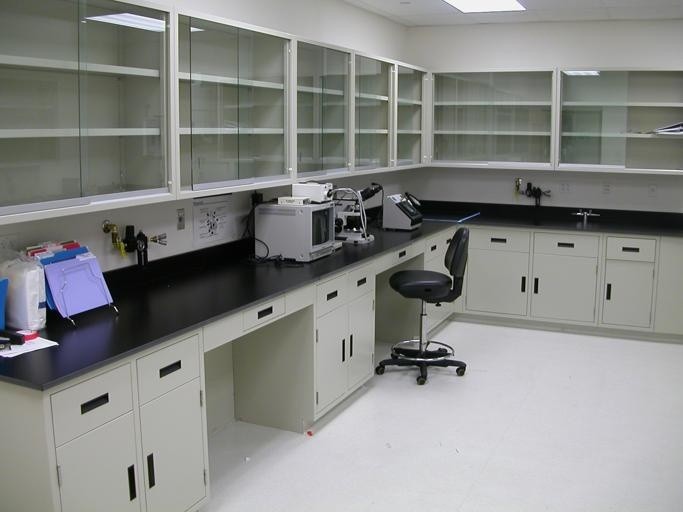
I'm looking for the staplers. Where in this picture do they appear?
[0,331,26,345]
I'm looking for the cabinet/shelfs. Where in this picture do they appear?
[0,0,426,223]
[427,69,682,178]
[461,202,681,342]
[0,200,461,512]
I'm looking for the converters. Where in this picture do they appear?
[277,196,311,206]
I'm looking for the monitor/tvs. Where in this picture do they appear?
[312,205,333,249]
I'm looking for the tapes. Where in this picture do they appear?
[15,330,39,340]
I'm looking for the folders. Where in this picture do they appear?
[26,239,114,318]
